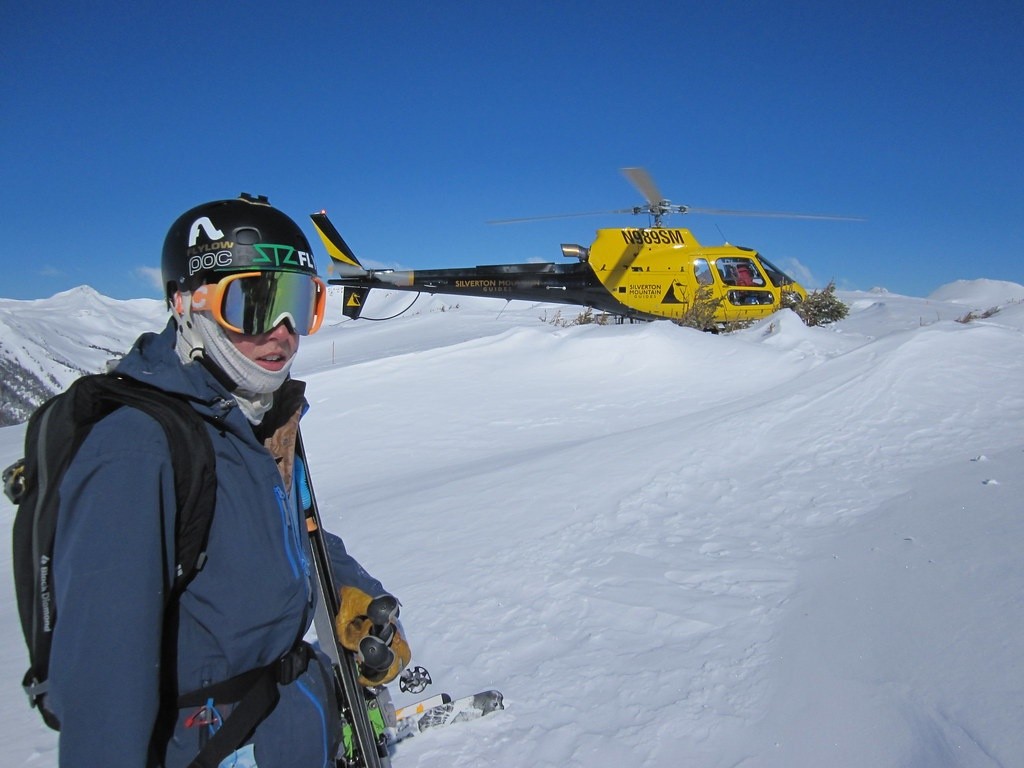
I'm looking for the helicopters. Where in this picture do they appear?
[310,165,869,336]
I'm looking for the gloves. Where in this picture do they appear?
[355,618,411,688]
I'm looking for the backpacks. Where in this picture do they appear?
[2,370,315,768]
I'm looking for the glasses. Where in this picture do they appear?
[173,263,330,336]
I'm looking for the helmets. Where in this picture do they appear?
[161,191,318,312]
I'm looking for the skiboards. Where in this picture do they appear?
[292,426,509,768]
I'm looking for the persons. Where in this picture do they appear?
[45,198,410,768]
[734,261,758,292]
